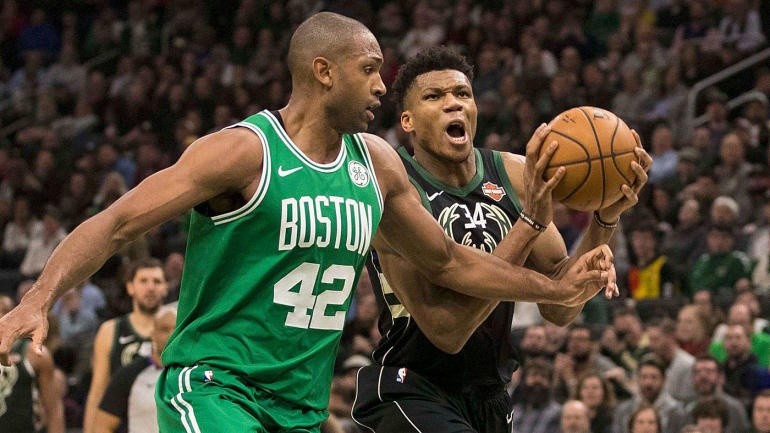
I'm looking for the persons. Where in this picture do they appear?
[0,0,770,433]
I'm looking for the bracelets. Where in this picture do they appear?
[519,212,547,232]
[594,210,620,228]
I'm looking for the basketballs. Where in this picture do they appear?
[536,106,639,213]
